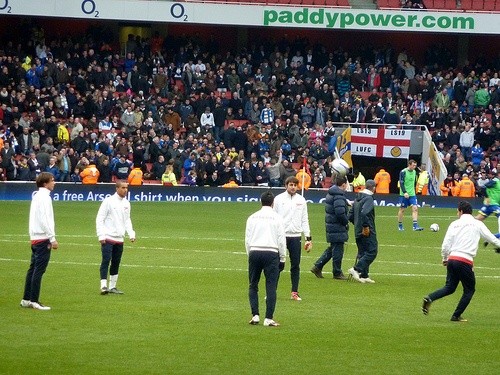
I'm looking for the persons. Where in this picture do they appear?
[96,179,136,295]
[264,176,313,301]
[0,24,500,201]
[79,161,100,184]
[422,198,500,321]
[374,166,392,195]
[126,161,143,185]
[20,172,59,310]
[310,174,349,280]
[161,164,177,185]
[244,191,287,327]
[473,169,500,247]
[397,159,425,231]
[346,179,379,283]
[456,0,461,8]
[399,0,427,11]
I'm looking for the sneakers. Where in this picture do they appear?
[263,318,280,327]
[20,299,51,310]
[100,286,109,295]
[109,288,123,294]
[249,315,260,325]
[291,292,302,301]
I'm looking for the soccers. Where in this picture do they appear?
[430,223,439,232]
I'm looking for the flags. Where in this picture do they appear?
[336,127,353,169]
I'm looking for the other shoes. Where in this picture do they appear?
[311,266,324,278]
[413,226,424,231]
[451,316,467,322]
[398,227,404,231]
[421,296,432,315]
[333,273,349,280]
[348,267,365,284]
[360,277,375,284]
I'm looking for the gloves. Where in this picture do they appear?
[362,227,370,237]
[304,240,312,252]
[279,262,285,272]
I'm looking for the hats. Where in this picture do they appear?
[365,179,379,187]
